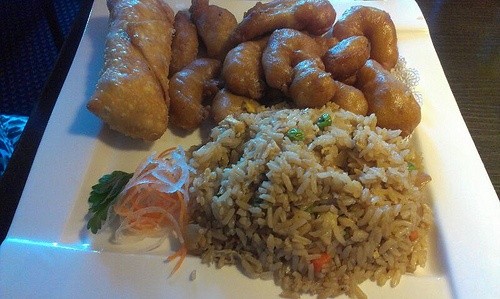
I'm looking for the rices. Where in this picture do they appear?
[186,103,435,299]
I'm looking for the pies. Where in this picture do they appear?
[85,0,174,138]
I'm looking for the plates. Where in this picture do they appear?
[1,0,500,299]
